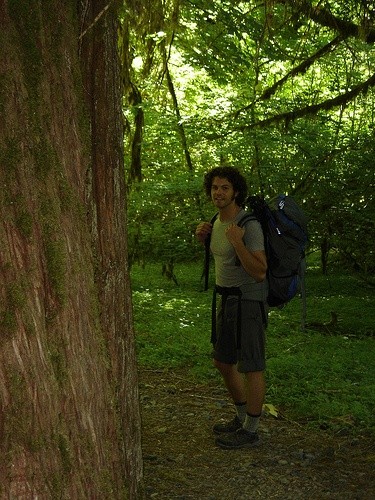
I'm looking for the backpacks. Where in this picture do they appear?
[207,194,308,307]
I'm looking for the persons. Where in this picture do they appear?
[188,158,272,451]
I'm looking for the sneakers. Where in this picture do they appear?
[213,416,242,434]
[218,429,259,449]
